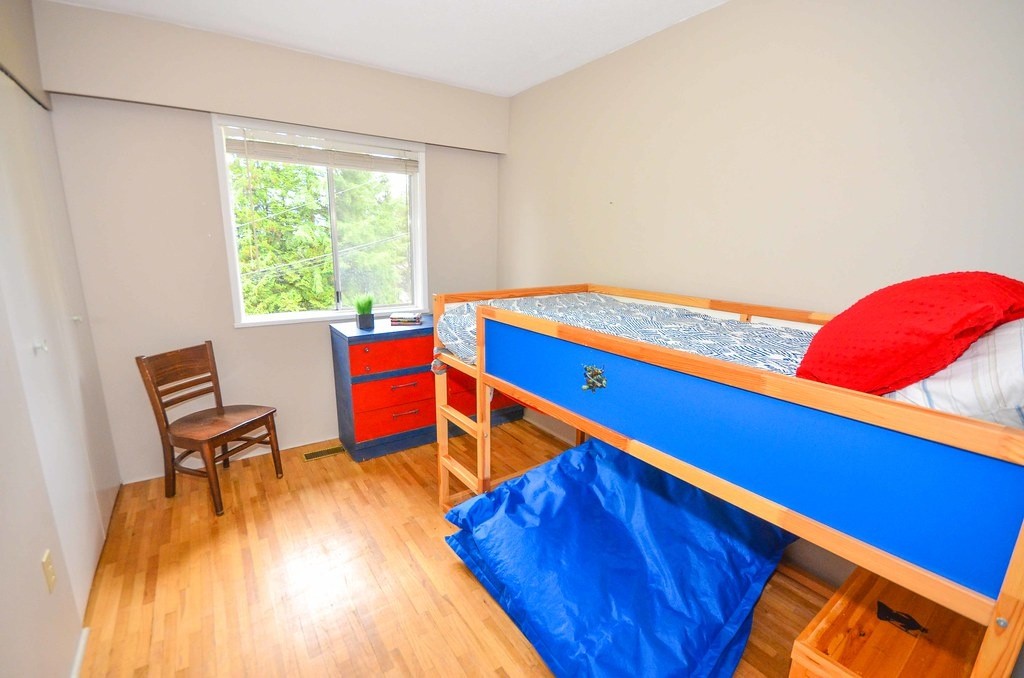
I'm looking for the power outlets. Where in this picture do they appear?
[41,549,59,595]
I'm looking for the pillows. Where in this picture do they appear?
[883,319,1024,430]
[796,270,1024,396]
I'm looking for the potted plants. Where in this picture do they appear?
[354,296,374,329]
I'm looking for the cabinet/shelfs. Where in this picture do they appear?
[329,314,525,463]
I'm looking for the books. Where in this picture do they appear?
[390,313,422,325]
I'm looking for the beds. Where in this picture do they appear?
[431,284,1024,678]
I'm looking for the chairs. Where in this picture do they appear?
[135,338,284,517]
